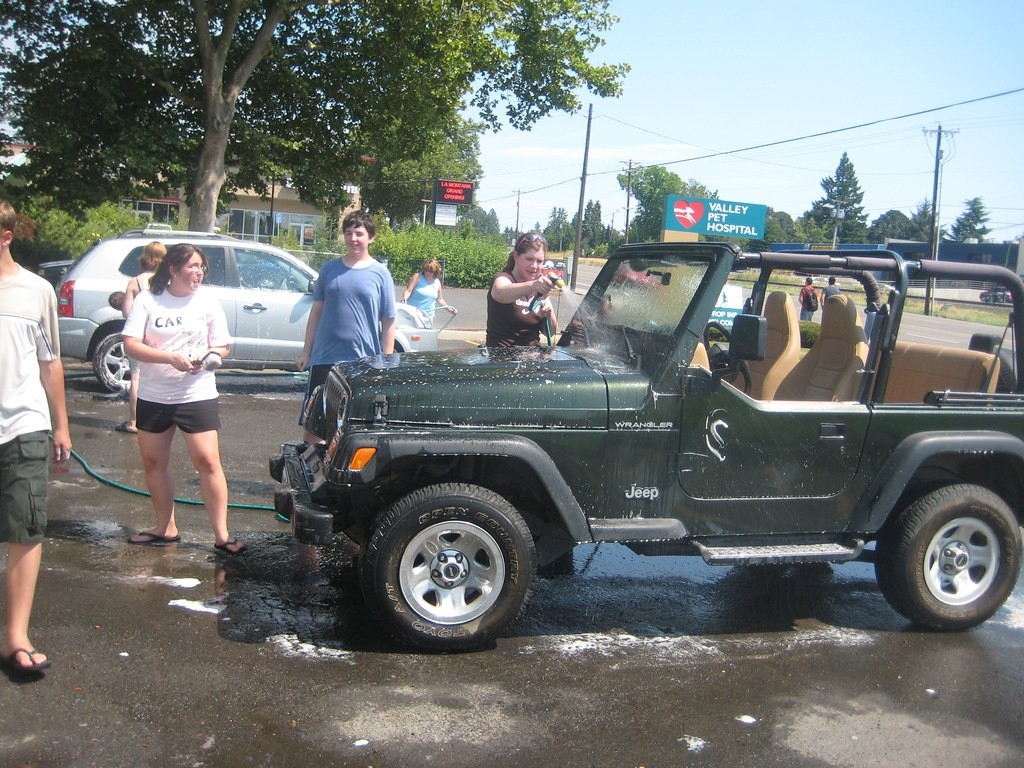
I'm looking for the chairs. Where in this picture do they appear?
[773,293,868,402]
[733,291,801,400]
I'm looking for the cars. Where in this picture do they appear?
[979,286,1014,304]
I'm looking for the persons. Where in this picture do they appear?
[295,211,397,442]
[403,259,458,314]
[486,232,558,346]
[0,198,72,673]
[121,243,248,554]
[799,276,841,320]
[108,241,166,432]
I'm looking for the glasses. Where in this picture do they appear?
[515,234,548,250]
[424,268,433,273]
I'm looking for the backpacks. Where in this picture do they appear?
[803,287,818,311]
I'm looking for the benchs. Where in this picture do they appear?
[883,341,1000,404]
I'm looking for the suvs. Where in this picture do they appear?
[269,240,1024,656]
[54,228,440,396]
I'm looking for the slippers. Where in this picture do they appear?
[214,537,247,555]
[115,422,137,433]
[127,531,180,543]
[1,648,51,672]
